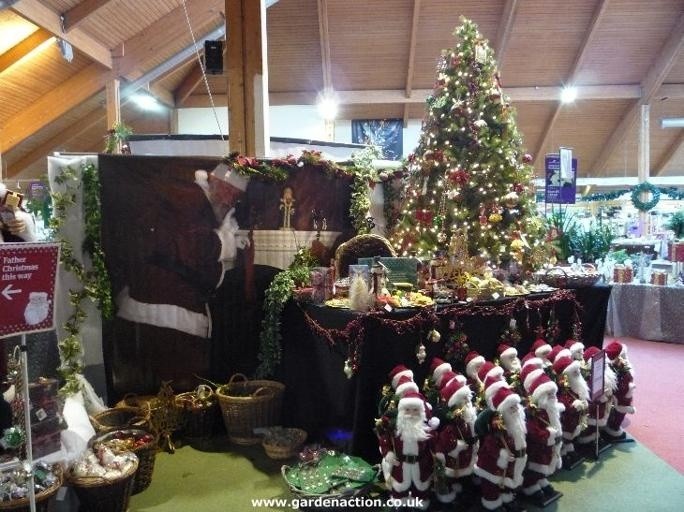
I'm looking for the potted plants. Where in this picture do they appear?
[579,221,598,270]
[539,200,578,266]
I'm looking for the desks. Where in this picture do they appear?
[299,272,612,459]
[604,275,684,345]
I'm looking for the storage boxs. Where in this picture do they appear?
[16,379,61,461]
[614,269,632,283]
[668,243,684,262]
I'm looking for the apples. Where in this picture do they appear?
[469,277,498,288]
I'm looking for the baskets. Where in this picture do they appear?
[333,234,418,284]
[466,278,505,299]
[540,263,601,288]
[0,373,383,512]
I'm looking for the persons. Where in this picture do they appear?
[0,181,39,243]
[378,337,636,512]
[112,151,251,392]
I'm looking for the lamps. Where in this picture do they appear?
[662,118,684,127]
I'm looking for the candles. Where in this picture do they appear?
[312,209,318,229]
[322,218,327,230]
